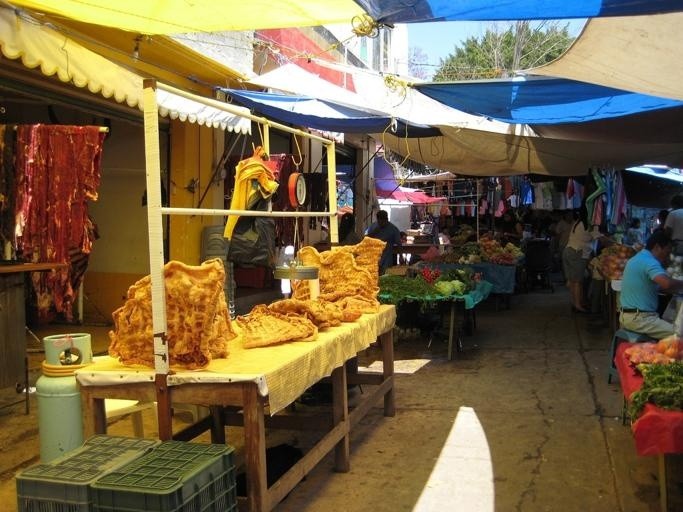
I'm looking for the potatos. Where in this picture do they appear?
[479,239,501,254]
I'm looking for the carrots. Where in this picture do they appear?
[488,252,514,265]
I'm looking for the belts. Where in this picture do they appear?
[620,308,637,313]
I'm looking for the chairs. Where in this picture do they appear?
[524,240,556,292]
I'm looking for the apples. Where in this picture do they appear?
[621,335,683,367]
[599,245,637,280]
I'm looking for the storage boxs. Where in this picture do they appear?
[93,439,239,511]
[14,434,161,512]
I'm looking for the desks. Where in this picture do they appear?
[614,341,683,512]
[393,243,455,266]
[377,278,493,360]
[605,278,622,345]
[75,303,397,511]
[418,255,526,312]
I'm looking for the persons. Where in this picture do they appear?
[493,196,622,313]
[438,215,496,245]
[619,196,683,340]
[341,210,408,276]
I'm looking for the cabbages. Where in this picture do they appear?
[434,281,455,298]
[451,279,467,296]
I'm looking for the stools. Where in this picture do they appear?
[607,328,659,384]
